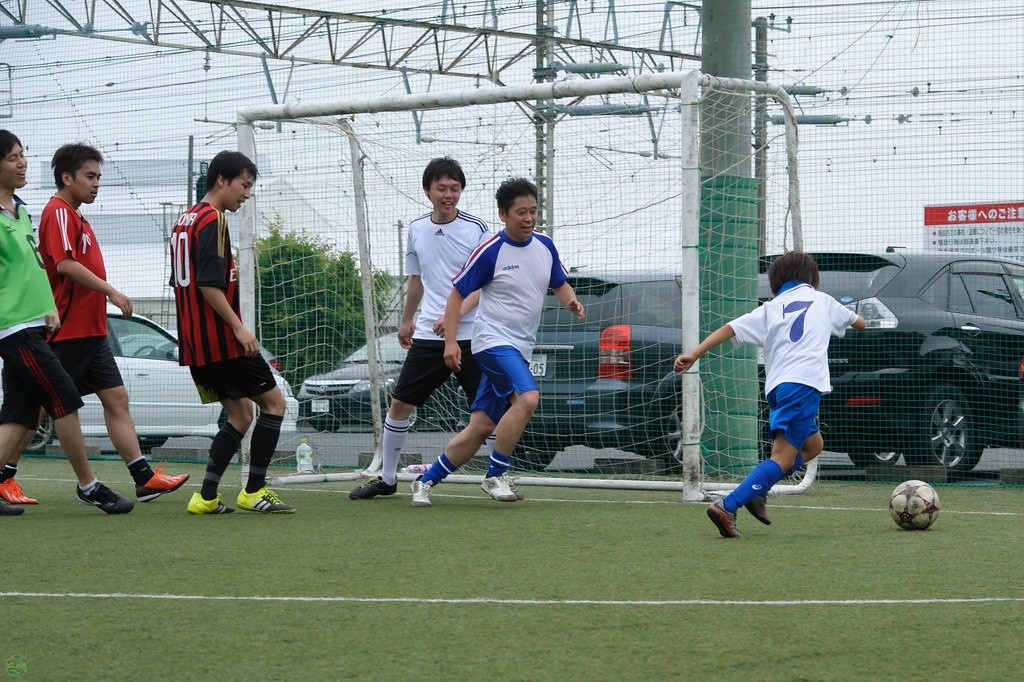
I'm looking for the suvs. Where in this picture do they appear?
[759,247,1024,480]
[498,266,686,474]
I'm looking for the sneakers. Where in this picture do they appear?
[500,473,525,500]
[0,498,24,515]
[135,472,190,502]
[410,474,433,508]
[237,485,295,514]
[77,481,134,514]
[349,474,397,499]
[745,496,771,525]
[706,498,742,538]
[186,492,235,515]
[480,472,517,501]
[0,478,40,505]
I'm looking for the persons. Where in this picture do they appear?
[672,253,864,537]
[0,129,135,515]
[0,143,190,504]
[407,179,586,508]
[169,150,296,513]
[349,157,523,500]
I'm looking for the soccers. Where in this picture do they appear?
[888,478,941,530]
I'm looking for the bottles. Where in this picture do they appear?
[296,439,313,474]
[401,464,432,473]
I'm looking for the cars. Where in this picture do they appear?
[298,330,467,429]
[0,302,298,457]
